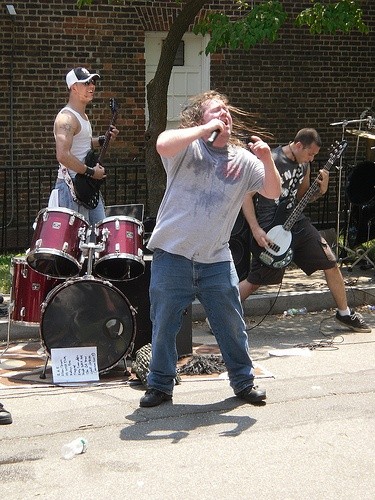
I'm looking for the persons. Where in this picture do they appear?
[139,89,281,408]
[53,67,119,224]
[206,128,372,336]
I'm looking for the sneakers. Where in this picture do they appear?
[334,309,372,333]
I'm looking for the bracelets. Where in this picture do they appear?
[84,165,96,177]
[98,135,105,145]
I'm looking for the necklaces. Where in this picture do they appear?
[289,140,298,164]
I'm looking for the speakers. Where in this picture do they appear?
[226,207,251,282]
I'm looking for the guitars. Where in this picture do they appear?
[250,140,348,270]
[75,98,119,210]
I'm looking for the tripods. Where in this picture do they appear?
[330,118,375,265]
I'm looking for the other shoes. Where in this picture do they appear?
[0,402,13,425]
[138,387,173,406]
[236,385,267,403]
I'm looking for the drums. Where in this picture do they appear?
[39,279,136,376]
[26,207,92,279]
[8,258,64,327]
[93,215,145,282]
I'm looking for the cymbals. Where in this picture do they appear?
[346,128,374,139]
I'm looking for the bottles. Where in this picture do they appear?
[61,438,87,460]
[283,307,307,316]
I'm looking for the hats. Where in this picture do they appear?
[66,67,101,91]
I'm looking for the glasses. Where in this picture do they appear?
[84,77,96,85]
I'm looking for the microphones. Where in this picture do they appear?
[76,91,87,97]
[208,128,220,143]
